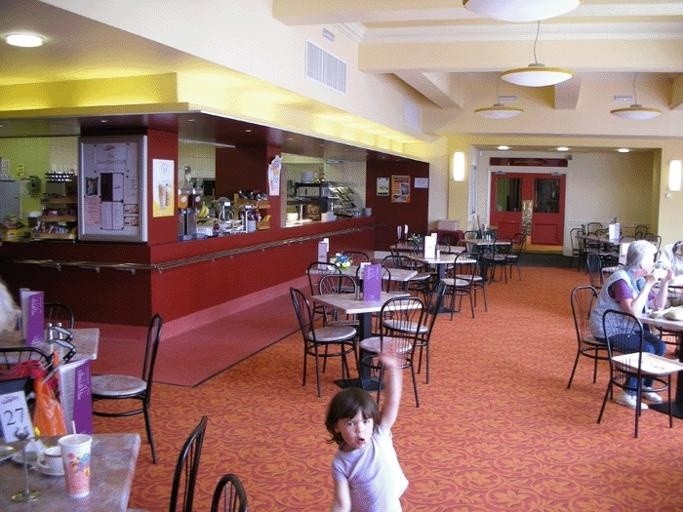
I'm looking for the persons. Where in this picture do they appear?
[87,179,97,194]
[325,356,410,512]
[590,240,671,411]
[672,241,683,276]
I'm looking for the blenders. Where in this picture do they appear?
[29,211,41,230]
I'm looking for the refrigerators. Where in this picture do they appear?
[0,176,20,219]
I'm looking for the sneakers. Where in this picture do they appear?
[614,386,661,413]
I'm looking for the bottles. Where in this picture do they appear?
[0,212,19,242]
[356,286,360,301]
[332,253,351,270]
[237,206,262,232]
[435,241,440,260]
[353,209,360,216]
[47,322,63,342]
[42,169,74,183]
[481,227,492,243]
[609,217,620,240]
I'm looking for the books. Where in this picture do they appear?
[20,291,45,344]
[609,223,620,242]
[364,264,381,302]
[317,241,327,270]
[58,359,93,435]
[424,236,435,258]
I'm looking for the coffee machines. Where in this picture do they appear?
[177,187,206,241]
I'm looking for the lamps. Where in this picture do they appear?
[609,72,663,120]
[464,1,582,24]
[453,151,465,181]
[669,159,683,191]
[474,73,522,122]
[4,32,46,48]
[501,31,572,89]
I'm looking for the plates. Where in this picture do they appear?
[362,208,372,216]
[39,466,65,476]
[664,311,683,322]
[301,171,313,183]
[0,445,49,465]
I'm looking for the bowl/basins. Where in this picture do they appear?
[287,213,298,221]
[649,309,663,319]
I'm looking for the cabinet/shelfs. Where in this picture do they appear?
[31,197,78,242]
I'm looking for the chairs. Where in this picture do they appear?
[567,222,683,440]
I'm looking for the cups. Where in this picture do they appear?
[58,433,93,498]
[38,446,64,473]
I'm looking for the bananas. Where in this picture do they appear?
[197,201,209,218]
